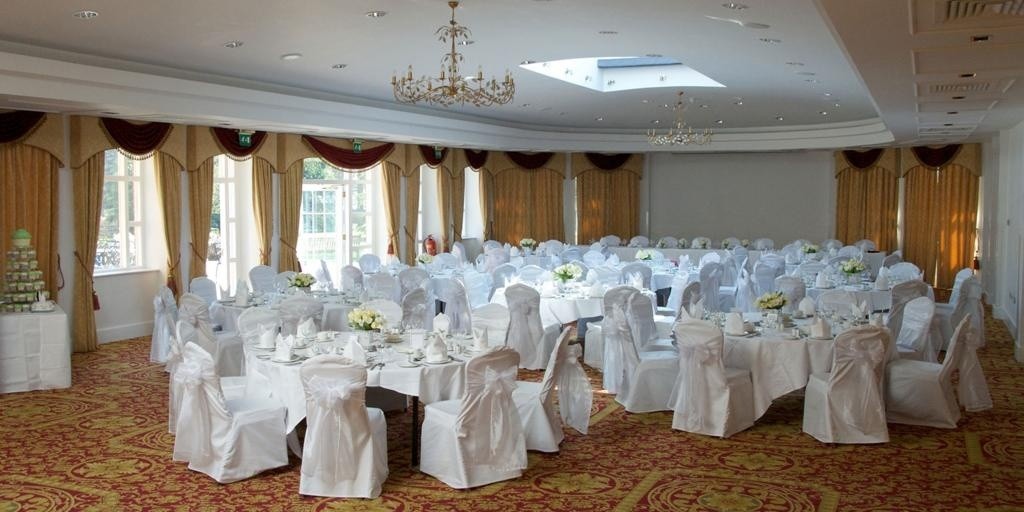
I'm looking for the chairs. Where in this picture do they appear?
[452,235,992,444]
[150,253,594,500]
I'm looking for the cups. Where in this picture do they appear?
[0,229,50,313]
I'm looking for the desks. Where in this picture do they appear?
[0,302,73,394]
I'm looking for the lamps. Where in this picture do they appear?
[647,91,713,146]
[388,1,516,109]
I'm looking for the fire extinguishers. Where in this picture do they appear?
[423,232,437,256]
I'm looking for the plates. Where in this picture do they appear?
[221,292,358,311]
[536,281,610,302]
[802,272,900,291]
[252,333,474,369]
[708,309,861,341]
